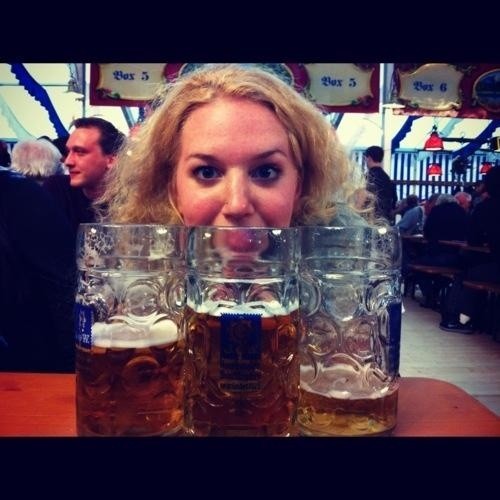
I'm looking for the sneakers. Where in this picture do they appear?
[439,318,474,334]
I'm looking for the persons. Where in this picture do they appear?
[392,164,500,344]
[362,146,397,228]
[0,117,130,372]
[87,62,380,329]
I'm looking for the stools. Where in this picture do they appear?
[398,263,500,341]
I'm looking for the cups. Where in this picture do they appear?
[298,221,399,436]
[188,227,305,438]
[78,224,184,439]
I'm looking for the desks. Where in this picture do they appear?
[0,372,500,436]
